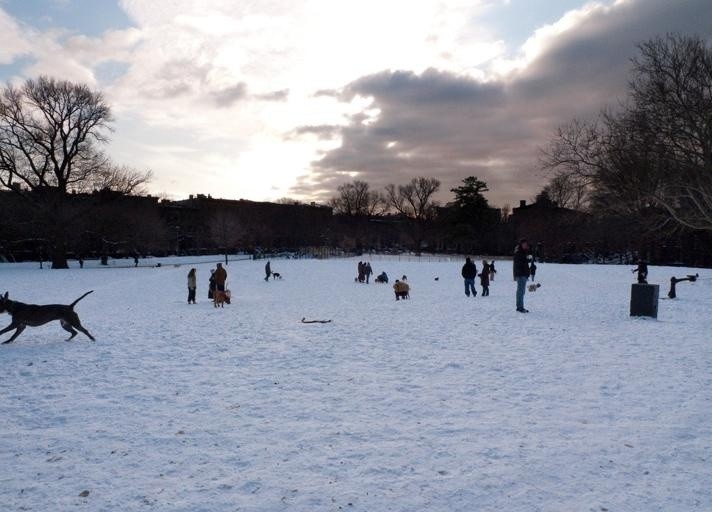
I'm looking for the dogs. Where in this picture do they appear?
[209,283,231,308]
[0,290,97,345]
[402,274,408,282]
[272,270,282,281]
[527,283,541,292]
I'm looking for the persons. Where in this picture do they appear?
[394,278,409,300]
[358,261,389,284]
[633,259,648,284]
[462,255,497,297]
[187,263,227,306]
[529,259,537,279]
[263,261,271,281]
[513,236,530,313]
